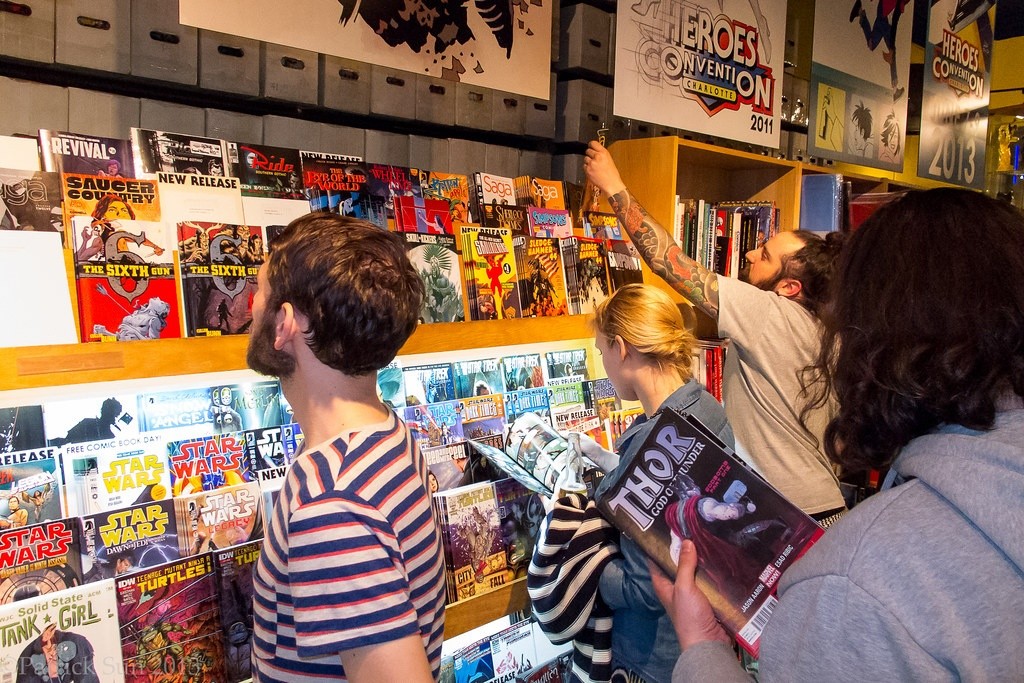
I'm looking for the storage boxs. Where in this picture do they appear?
[0,0,814,183]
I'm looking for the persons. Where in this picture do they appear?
[248,206,448,683]
[581,140,849,530]
[651,189,1024,683]
[537,283,734,682]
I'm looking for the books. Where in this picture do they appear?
[0,129,901,683]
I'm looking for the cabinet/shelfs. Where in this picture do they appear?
[1,133,976,683]
